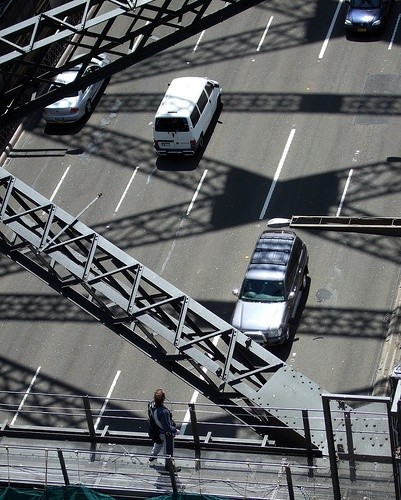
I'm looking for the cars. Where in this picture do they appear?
[344,0,387,40]
[44,53,105,124]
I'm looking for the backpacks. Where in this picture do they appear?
[148,402,171,445]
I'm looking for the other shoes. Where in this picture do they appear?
[165,465,181,473]
[149,455,158,462]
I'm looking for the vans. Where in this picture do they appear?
[153,75,222,158]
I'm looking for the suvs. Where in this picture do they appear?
[229,230,310,348]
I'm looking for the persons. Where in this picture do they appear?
[145,389,181,472]
